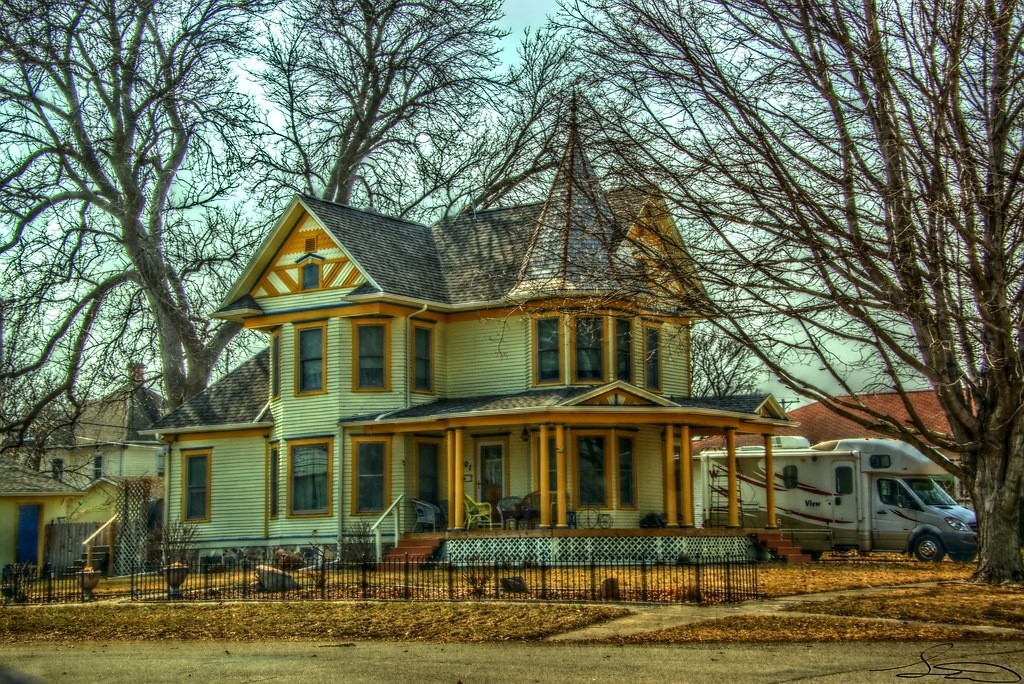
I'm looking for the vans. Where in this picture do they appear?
[701,436,981,564]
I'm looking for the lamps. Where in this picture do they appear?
[660,429,666,442]
[521,425,530,442]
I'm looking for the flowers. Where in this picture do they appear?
[84,566,94,572]
[170,561,187,568]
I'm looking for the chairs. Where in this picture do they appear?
[410,497,449,532]
[463,493,493,531]
[497,491,565,530]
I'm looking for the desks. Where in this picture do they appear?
[503,511,540,530]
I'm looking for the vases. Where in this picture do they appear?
[77,571,102,599]
[162,567,189,596]
